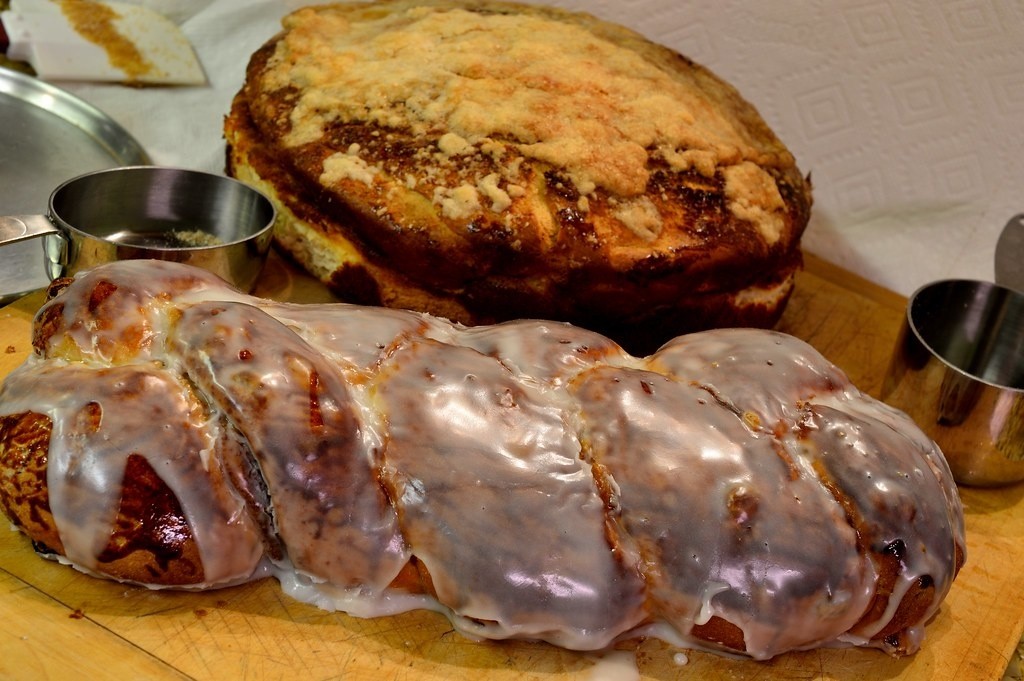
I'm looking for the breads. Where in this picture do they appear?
[221,0,820,359]
[0,258,971,657]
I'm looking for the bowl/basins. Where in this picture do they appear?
[881,279,1024,488]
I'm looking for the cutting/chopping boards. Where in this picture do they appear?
[0,247,1024,681]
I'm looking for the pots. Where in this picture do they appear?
[0,164,278,295]
